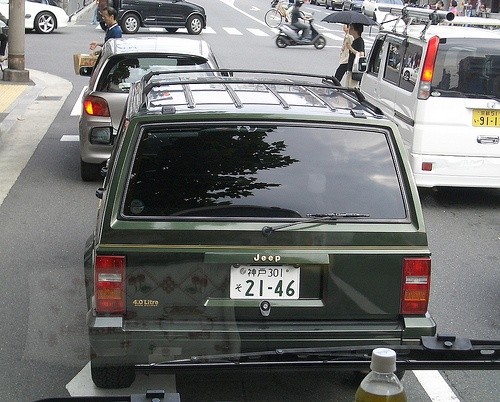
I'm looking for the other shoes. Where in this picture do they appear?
[301,38,312,43]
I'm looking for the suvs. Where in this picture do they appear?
[95,0,206,35]
[78,37,234,182]
[83,66,434,391]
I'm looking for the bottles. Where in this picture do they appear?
[354,347,407,402]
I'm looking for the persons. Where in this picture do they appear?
[0,12,7,61]
[335,23,366,88]
[285,0,294,22]
[401,0,490,26]
[90,6,122,51]
[291,0,311,40]
[90,0,107,25]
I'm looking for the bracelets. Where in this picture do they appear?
[351,49,354,53]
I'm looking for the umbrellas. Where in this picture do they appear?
[321,8,378,41]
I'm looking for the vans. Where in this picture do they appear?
[357,20,500,190]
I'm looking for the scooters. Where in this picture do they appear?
[271,14,327,50]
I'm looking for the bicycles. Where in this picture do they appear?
[265,2,304,27]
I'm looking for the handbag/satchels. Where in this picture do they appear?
[73,49,99,75]
[339,71,359,88]
[352,51,367,74]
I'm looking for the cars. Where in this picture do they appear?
[361,0,407,21]
[0,0,70,35]
[288,0,364,12]
